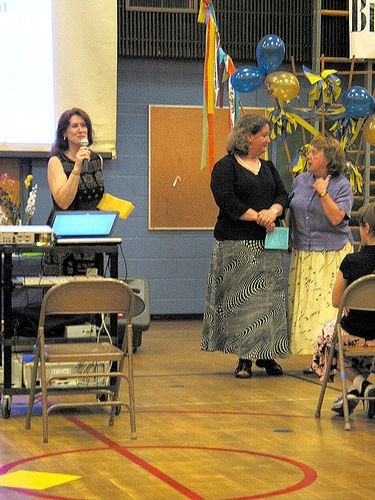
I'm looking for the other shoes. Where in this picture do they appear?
[255,358,283,375]
[234,359,252,379]
[359,380,375,419]
[330,389,360,415]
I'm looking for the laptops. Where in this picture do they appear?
[51,211,122,243]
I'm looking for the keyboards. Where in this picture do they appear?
[20,273,104,285]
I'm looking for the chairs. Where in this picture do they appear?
[23,277,138,444]
[313,273,375,429]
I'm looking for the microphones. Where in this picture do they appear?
[80,139,88,171]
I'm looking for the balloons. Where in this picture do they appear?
[231,65,265,93]
[265,71,299,101]
[364,112,375,147]
[256,34,285,74]
[342,86,375,118]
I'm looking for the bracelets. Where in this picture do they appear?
[319,190,328,197]
[270,207,278,216]
[72,169,81,176]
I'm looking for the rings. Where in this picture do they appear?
[268,217,270,221]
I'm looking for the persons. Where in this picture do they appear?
[42,108,105,300]
[201,114,289,378]
[311,202,375,417]
[289,132,356,384]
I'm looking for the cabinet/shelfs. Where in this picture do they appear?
[0,237,124,416]
